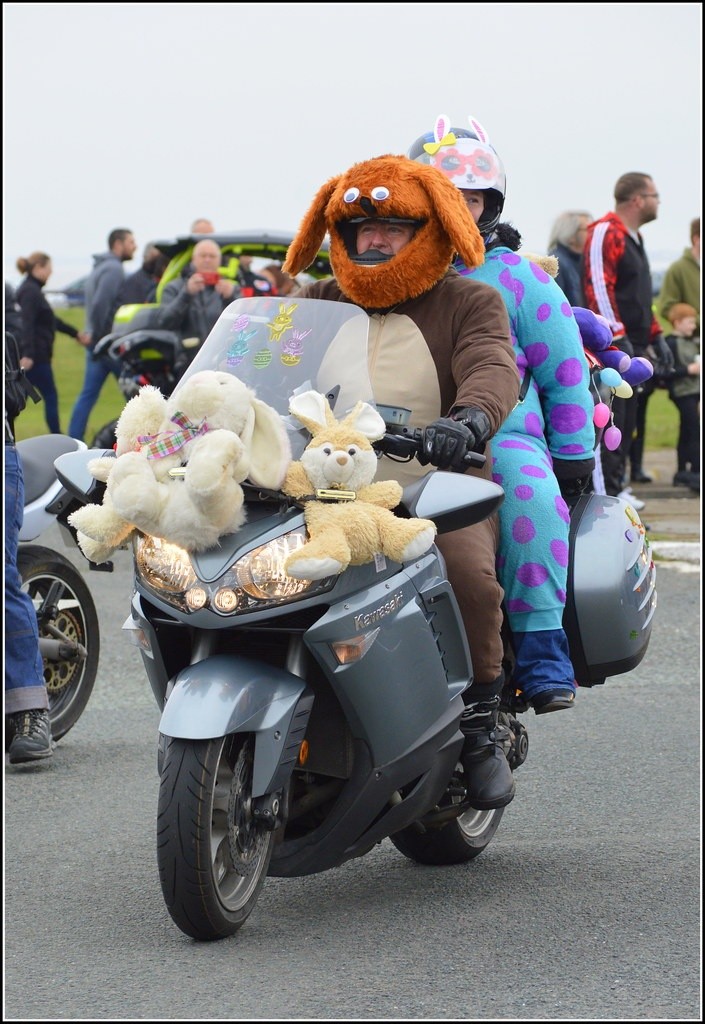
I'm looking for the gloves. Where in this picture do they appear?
[611,337,635,360]
[557,471,589,501]
[416,406,494,470]
[650,333,674,373]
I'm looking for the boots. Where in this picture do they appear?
[460,668,516,810]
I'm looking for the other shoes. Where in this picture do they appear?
[630,471,652,485]
[537,690,573,714]
[673,462,700,492]
[618,488,644,513]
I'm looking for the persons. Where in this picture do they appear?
[4,252,90,433]
[547,173,701,510]
[5,411,56,764]
[68,217,296,440]
[219,124,591,811]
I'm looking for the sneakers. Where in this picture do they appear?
[10,704,53,764]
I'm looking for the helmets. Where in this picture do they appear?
[346,215,416,269]
[407,124,508,235]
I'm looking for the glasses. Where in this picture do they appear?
[636,191,658,202]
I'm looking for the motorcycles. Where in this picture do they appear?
[51,296,657,941]
[1,435,113,755]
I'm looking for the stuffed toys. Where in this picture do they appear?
[69,386,168,562]
[109,369,292,551]
[282,391,437,581]
[569,306,653,387]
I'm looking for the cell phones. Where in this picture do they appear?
[199,271,219,285]
